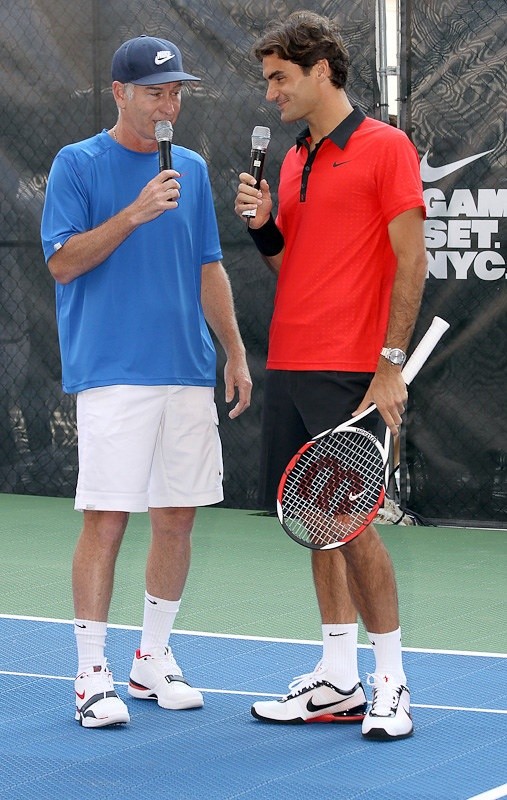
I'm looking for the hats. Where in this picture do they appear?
[110,34,202,86]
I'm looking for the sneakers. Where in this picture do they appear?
[71,662,133,729]
[360,669,413,739]
[249,657,367,722]
[129,649,206,711]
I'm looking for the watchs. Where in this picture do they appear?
[380,347,407,366]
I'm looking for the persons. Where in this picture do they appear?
[41,34,253,728]
[234,11,429,742]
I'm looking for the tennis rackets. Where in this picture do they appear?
[275,316,451,551]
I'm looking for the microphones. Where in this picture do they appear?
[154,120,175,202]
[242,126,272,218]
